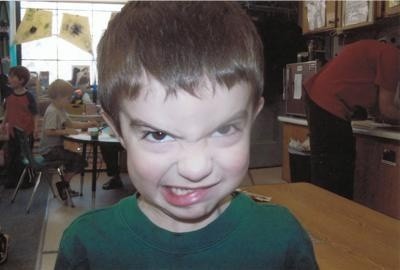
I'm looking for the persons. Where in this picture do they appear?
[302,40,400,198]
[0,66,126,201]
[52,0,317,270]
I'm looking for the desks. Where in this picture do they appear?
[239,182,399,269]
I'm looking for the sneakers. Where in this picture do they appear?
[102,176,122,189]
[0,234,9,263]
[56,180,79,199]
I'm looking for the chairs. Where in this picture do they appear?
[11,86,120,213]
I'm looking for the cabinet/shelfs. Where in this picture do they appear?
[297,0,400,36]
[282,122,399,221]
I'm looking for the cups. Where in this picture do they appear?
[90,128,99,139]
